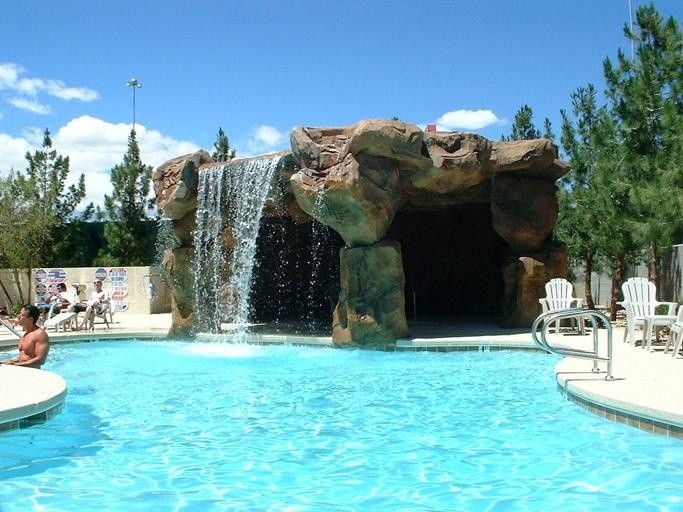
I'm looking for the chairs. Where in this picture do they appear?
[538,275,584,335]
[615,275,683,358]
[39,296,112,331]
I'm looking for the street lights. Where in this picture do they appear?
[126,78,141,130]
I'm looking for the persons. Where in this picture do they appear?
[3,303,49,369]
[67,278,110,331]
[36,282,72,316]
